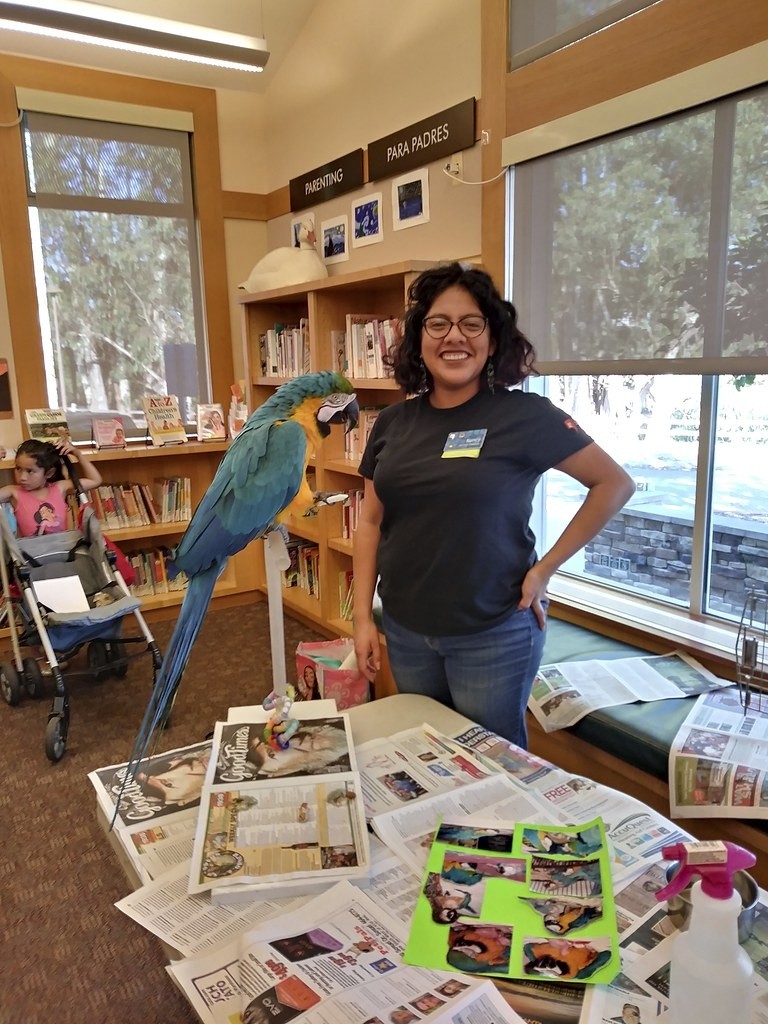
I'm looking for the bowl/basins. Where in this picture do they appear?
[665,862,760,943]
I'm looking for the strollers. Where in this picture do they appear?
[0,457,171,764]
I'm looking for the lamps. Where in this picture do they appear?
[0,0,271,73]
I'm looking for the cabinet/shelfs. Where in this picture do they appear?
[230,261,489,700]
[0,437,262,633]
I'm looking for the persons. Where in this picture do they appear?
[420,752,438,761]
[218,723,348,778]
[0,437,102,676]
[111,744,212,810]
[204,411,224,432]
[354,262,635,752]
[112,429,123,444]
[441,979,470,996]
[416,995,445,1012]
[163,419,182,430]
[385,771,427,800]
[611,1003,641,1024]
[304,665,321,701]
[391,1005,421,1024]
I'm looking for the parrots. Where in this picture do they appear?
[97,371,362,834]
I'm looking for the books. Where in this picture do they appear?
[92,417,125,449]
[24,408,72,446]
[143,396,188,447]
[196,404,226,442]
[84,476,192,530]
[121,542,189,597]
[260,314,406,622]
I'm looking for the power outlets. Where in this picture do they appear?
[451,152,464,186]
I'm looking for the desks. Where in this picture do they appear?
[97,691,768,1024]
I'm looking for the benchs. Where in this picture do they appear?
[523,615,768,891]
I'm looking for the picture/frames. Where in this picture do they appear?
[392,167,431,233]
[321,214,349,266]
[350,192,383,248]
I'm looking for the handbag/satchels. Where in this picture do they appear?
[295,638,371,711]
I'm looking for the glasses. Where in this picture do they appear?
[422,315,488,339]
